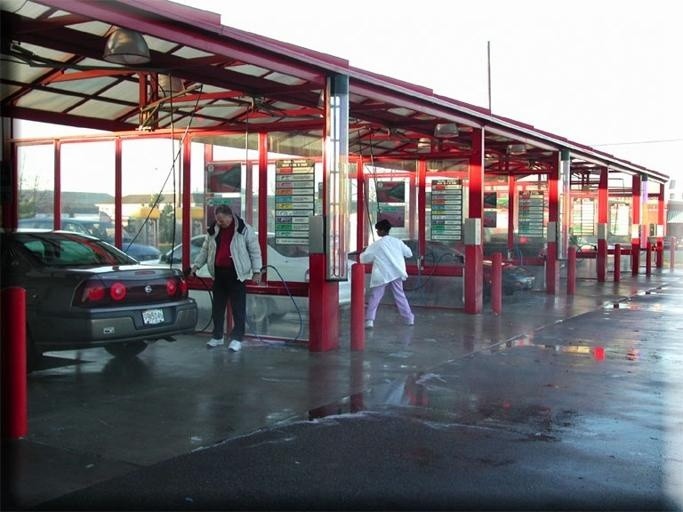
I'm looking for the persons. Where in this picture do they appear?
[359,219,414,329]
[189,204,262,351]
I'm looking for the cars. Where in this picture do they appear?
[575,238,618,258]
[346,237,538,300]
[137,227,367,331]
[16,215,161,262]
[662,235,678,250]
[16,226,198,364]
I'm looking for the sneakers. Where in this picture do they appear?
[404,319,414,326]
[206,335,224,348]
[364,318,374,329]
[227,339,241,352]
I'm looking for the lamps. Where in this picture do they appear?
[101,27,150,65]
[505,144,526,155]
[433,123,458,138]
